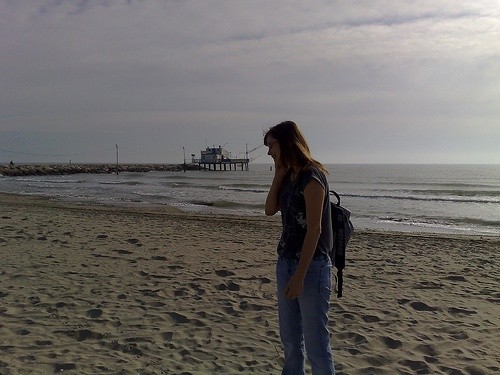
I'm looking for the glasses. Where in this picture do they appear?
[267,141,278,148]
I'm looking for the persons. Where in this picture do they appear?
[263,121,340,375]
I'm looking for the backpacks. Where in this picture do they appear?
[328,190,354,270]
